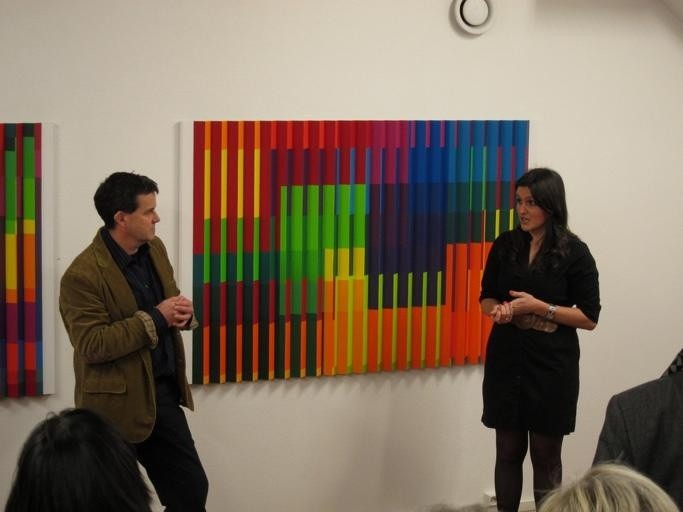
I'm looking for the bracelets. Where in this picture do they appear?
[542,303,557,322]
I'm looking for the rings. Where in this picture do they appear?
[505,318,510,320]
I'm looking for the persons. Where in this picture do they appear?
[588,349,683,512]
[4,407,152,512]
[478,168,601,512]
[58,173,209,512]
[533,462,682,512]
